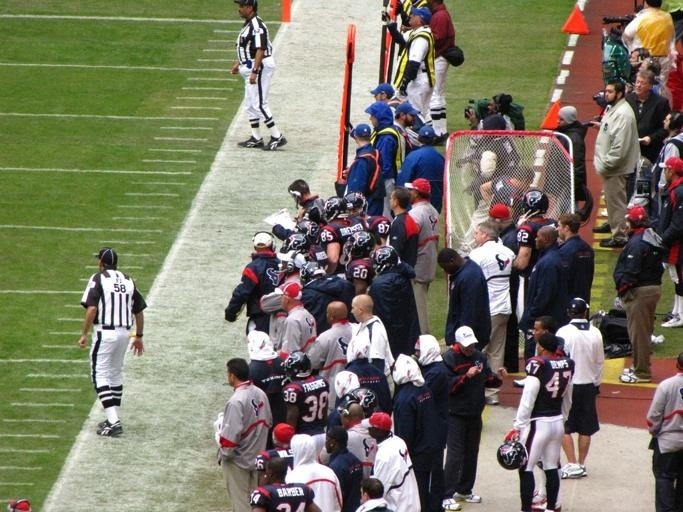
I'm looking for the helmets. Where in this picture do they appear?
[521,189,549,217]
[280,191,399,286]
[283,351,312,380]
[564,296,590,318]
[353,387,377,414]
[497,440,528,471]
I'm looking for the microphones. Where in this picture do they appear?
[295,199,300,209]
[274,269,287,273]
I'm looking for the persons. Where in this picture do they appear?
[380,0,455,146]
[230,0,288,150]
[78,247,146,438]
[218,79,683,512]
[602,1,682,165]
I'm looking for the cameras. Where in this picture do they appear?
[593,90,604,103]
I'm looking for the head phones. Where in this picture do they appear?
[289,188,303,202]
[343,399,358,416]
[629,48,645,62]
[287,249,302,273]
[669,114,683,129]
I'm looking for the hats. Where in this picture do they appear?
[234,1,258,7]
[628,204,647,222]
[559,105,577,124]
[271,422,294,448]
[540,332,557,350]
[412,6,431,24]
[454,325,480,349]
[489,203,511,221]
[353,83,439,195]
[254,233,273,249]
[361,411,391,431]
[92,248,118,266]
[658,157,683,172]
[274,281,303,301]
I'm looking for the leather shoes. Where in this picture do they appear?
[592,222,628,247]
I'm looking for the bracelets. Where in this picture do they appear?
[136,333,142,338]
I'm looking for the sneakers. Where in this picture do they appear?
[661,314,682,329]
[237,134,288,151]
[512,377,529,387]
[96,420,123,436]
[620,367,650,384]
[530,462,588,511]
[442,493,482,508]
